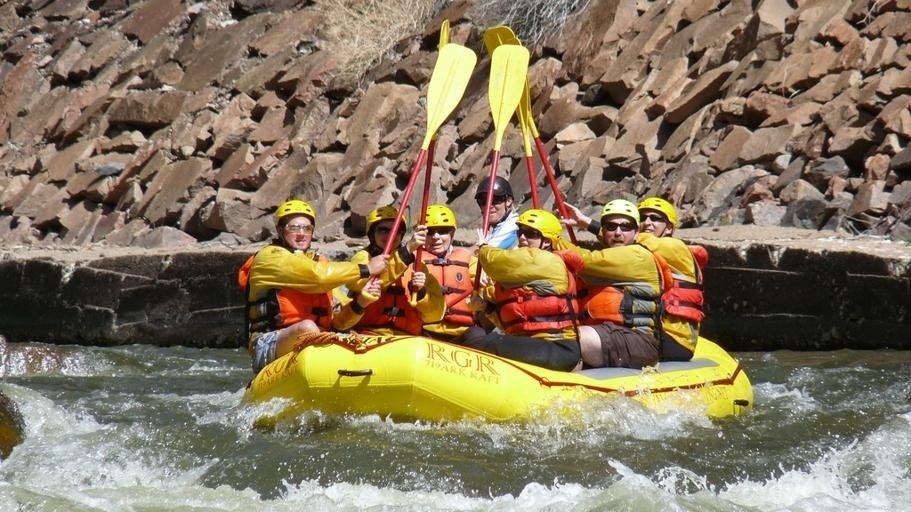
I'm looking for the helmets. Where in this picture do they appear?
[515,209,563,250]
[366,207,406,245]
[474,176,513,196]
[600,199,641,227]
[637,197,678,228]
[414,204,457,230]
[272,200,316,226]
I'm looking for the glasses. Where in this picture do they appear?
[477,196,506,208]
[603,221,636,231]
[377,225,402,235]
[517,230,538,239]
[427,227,451,236]
[285,225,313,234]
[640,214,665,222]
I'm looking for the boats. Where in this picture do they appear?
[237,333,754,432]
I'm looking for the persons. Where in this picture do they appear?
[239,200,493,373]
[473,175,709,374]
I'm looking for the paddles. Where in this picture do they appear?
[470,44,531,301]
[372,43,479,296]
[410,19,451,307]
[481,24,578,250]
